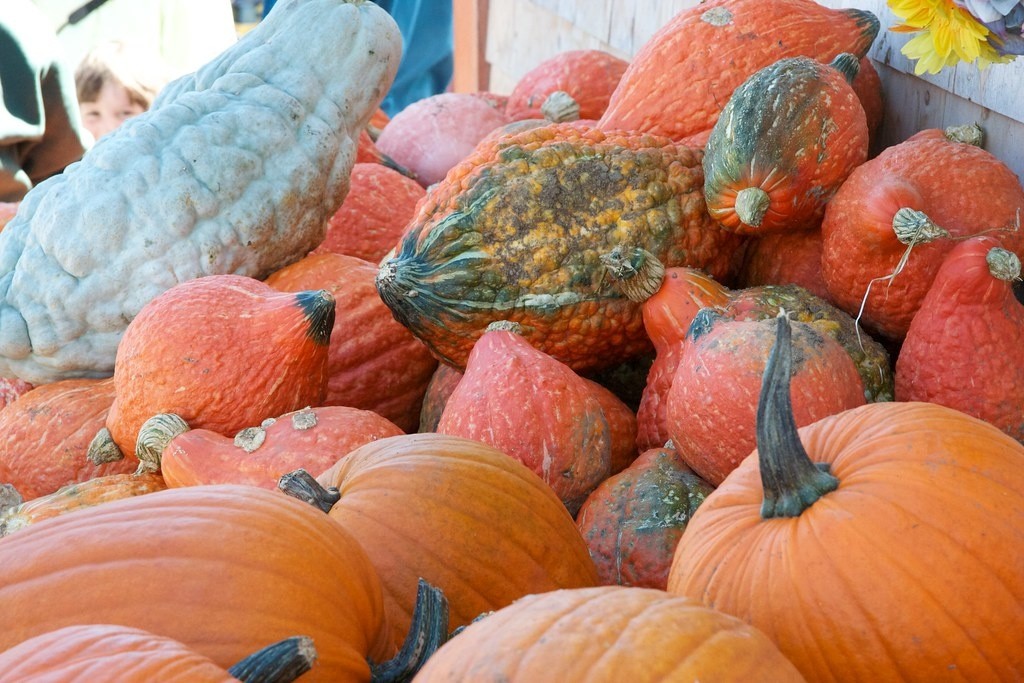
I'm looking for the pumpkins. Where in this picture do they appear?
[0,0,1024,683]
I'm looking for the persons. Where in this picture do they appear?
[69,35,165,152]
